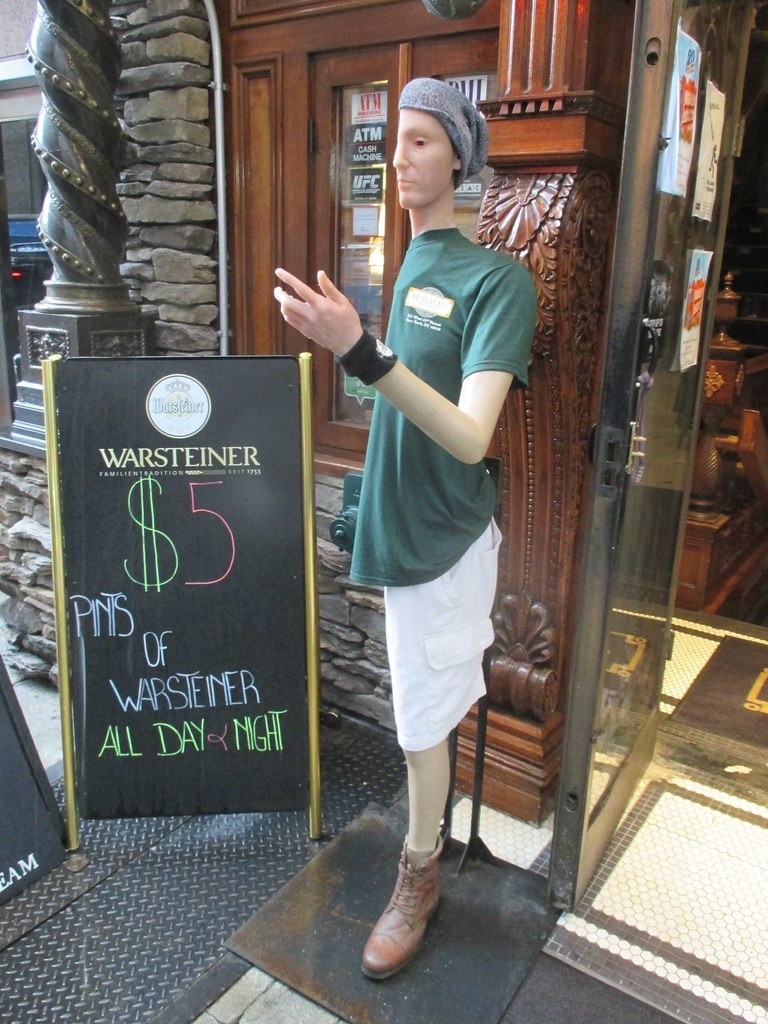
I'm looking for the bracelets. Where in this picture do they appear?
[335,331,399,386]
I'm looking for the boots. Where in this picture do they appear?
[359,833,446,982]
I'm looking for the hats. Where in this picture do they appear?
[395,75,489,191]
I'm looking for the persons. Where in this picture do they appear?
[273,77,539,978]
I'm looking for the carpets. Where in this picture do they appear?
[669,636,768,751]
